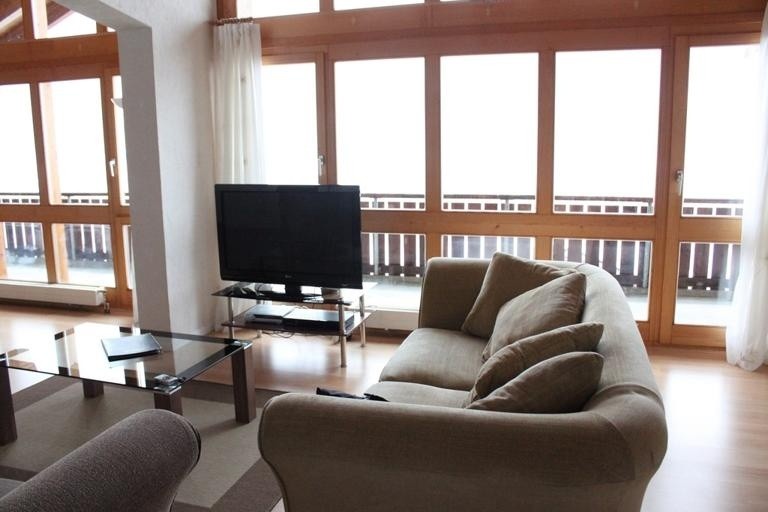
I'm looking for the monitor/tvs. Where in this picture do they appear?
[214,184,363,302]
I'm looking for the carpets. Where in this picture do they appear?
[0,373,284,512]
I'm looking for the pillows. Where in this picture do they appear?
[461,252,577,339]
[463,323,603,405]
[470,350,603,411]
[481,272,587,361]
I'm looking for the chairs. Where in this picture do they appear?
[0,409,201,512]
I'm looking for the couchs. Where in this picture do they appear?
[258,256,668,512]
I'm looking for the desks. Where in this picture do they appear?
[212,281,377,368]
